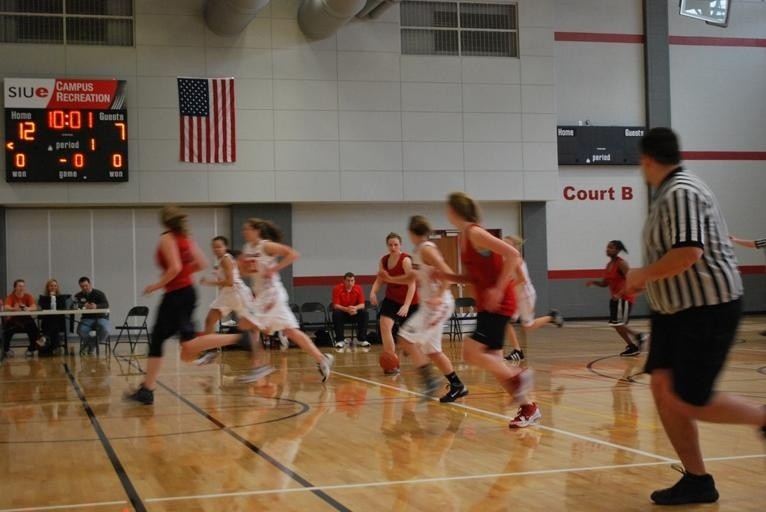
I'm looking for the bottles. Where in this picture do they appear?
[50,296,57,311]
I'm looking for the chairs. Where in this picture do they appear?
[114,355,147,381]
[301,302,330,345]
[361,303,379,336]
[270,303,301,349]
[327,300,370,345]
[113,305,150,354]
[453,297,477,342]
[37,294,73,350]
[78,324,109,355]
[218,314,263,351]
[442,312,460,342]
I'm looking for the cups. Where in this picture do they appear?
[73,300,79,310]
[81,298,87,310]
[20,304,25,311]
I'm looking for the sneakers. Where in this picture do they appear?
[621,346,640,356]
[549,308,563,327]
[384,349,541,428]
[635,332,647,345]
[129,332,371,405]
[651,463,719,505]
[5,336,96,357]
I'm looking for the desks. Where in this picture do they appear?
[0,309,110,353]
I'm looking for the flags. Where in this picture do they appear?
[178,76,236,164]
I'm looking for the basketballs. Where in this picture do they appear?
[381,351,399,371]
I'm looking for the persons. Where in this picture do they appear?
[0,277,112,356]
[379,215,468,403]
[728,235,766,336]
[502,234,563,362]
[586,239,650,357]
[370,233,420,375]
[122,207,260,404]
[627,127,766,506]
[197,236,255,368]
[237,219,335,384]
[331,272,372,347]
[430,193,541,427]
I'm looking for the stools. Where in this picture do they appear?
[2,329,35,354]
